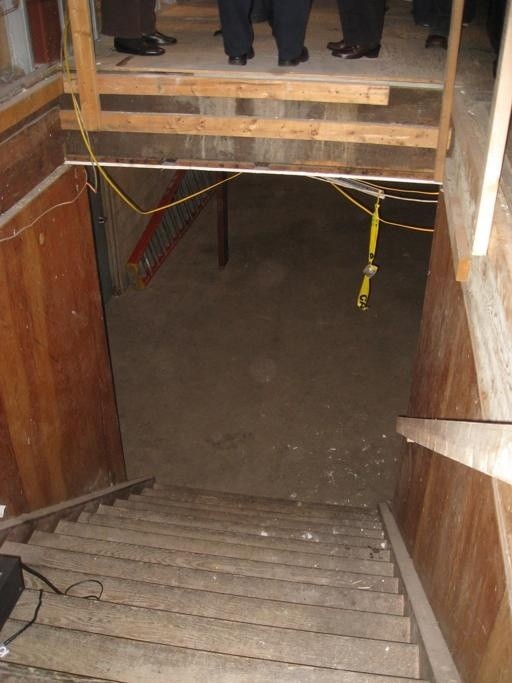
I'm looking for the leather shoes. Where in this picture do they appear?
[326,38,351,50]
[144,31,177,45]
[113,36,165,57]
[423,33,448,50]
[228,46,255,66]
[277,45,310,67]
[330,43,380,59]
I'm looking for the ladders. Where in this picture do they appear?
[126,169,217,291]
